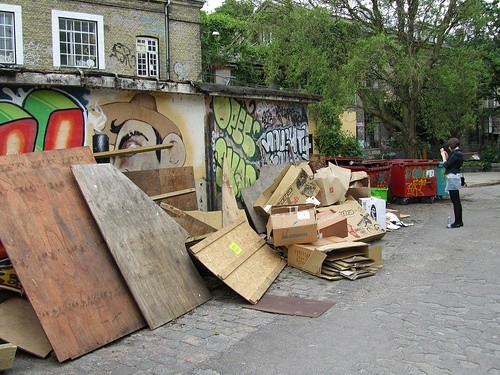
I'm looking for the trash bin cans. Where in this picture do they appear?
[321,157,365,172]
[435,162,449,200]
[362,160,393,207]
[391,159,439,204]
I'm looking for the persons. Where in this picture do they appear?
[441,138,464,228]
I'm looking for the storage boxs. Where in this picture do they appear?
[252,161,403,283]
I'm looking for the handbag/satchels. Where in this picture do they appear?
[461,176,468,187]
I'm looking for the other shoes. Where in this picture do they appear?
[446,222,463,228]
